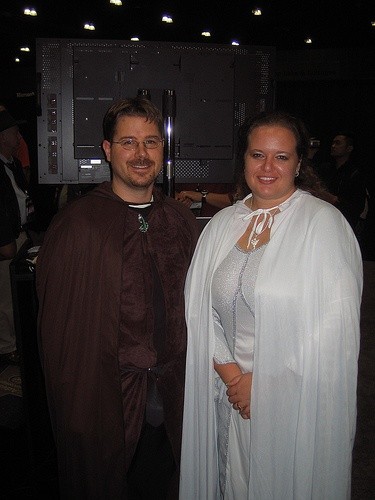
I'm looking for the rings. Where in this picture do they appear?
[237,401,243,411]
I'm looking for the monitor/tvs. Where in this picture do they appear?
[34,36,278,184]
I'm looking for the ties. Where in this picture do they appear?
[2,160,27,194]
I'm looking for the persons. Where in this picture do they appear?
[353,190,370,259]
[309,132,365,229]
[174,109,366,500]
[0,112,31,364]
[35,96,200,500]
[0,99,30,186]
[175,150,313,208]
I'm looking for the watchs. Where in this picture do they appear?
[200,189,209,207]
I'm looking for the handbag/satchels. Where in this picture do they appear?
[348,170,370,220]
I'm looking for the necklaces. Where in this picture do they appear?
[246,209,278,249]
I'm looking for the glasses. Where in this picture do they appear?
[109,138,164,149]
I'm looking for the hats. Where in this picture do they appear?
[0,110,27,132]
